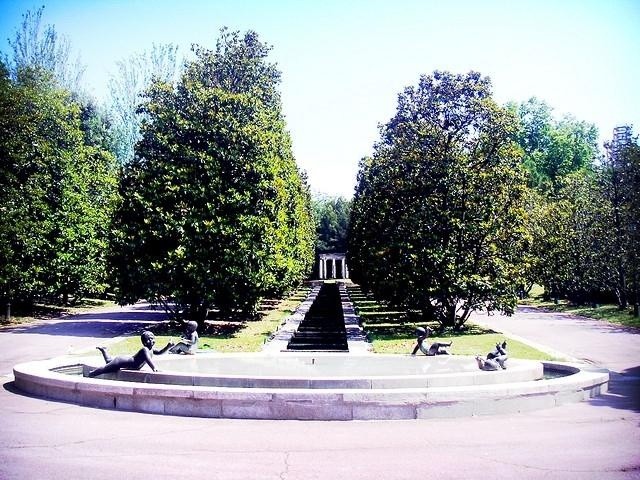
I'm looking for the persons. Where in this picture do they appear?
[411,326,453,355]
[89,330,175,377]
[169,320,199,354]
[475,340,509,370]
